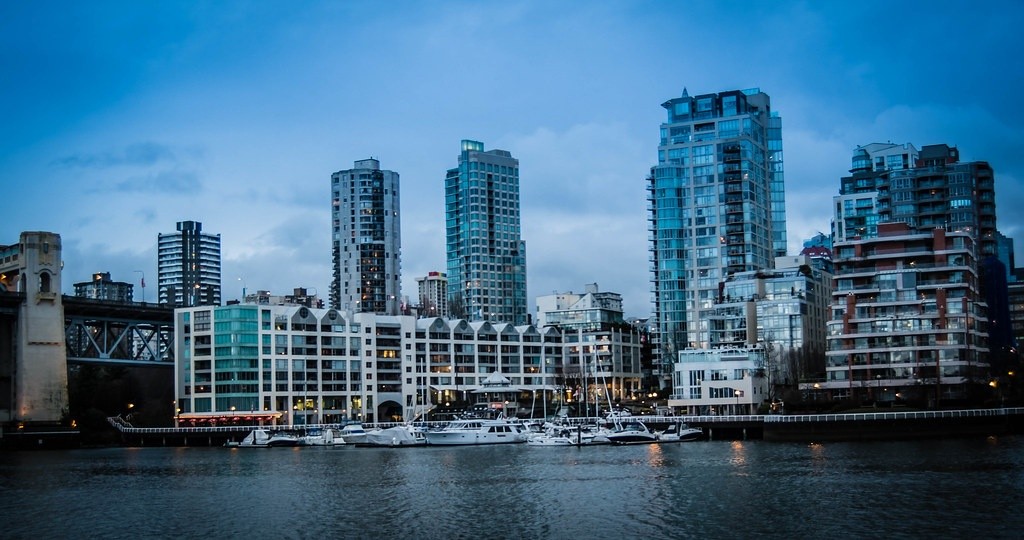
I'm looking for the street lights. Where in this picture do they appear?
[134,270,144,302]
[653,393,657,417]
[735,390,740,415]
[877,375,881,401]
[177,409,181,421]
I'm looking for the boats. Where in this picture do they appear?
[216,345,703,449]
